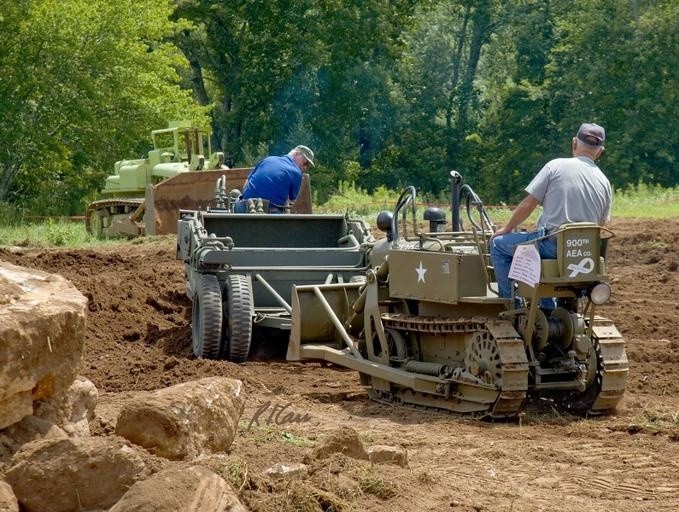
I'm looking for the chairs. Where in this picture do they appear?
[232,198,296,215]
[512,222,616,299]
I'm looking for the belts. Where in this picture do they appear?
[239,198,279,212]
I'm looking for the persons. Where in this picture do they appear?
[489,123,612,310]
[231,145,315,214]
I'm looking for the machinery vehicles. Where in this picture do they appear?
[174,175,378,364]
[284,169,631,422]
[83,126,312,241]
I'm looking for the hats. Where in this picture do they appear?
[296,145,315,168]
[577,123,606,146]
